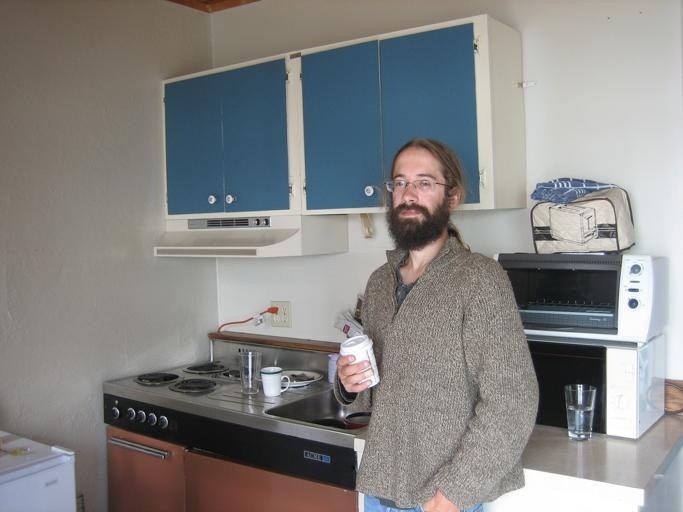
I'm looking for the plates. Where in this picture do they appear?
[280,370,324,388]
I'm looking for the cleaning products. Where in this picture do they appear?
[327,352,341,386]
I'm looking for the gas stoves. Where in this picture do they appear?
[103,361,243,447]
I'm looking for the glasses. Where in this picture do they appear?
[384,181,451,194]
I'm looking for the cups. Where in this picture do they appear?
[339,335,380,389]
[238,351,262,395]
[564,383,597,442]
[327,353,339,384]
[260,366,291,396]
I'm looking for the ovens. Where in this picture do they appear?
[492,251,669,344]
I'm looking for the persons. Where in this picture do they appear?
[333,138,541,512]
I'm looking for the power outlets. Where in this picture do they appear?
[271,300,293,327]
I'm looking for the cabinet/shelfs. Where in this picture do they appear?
[162,53,301,220]
[301,14,527,216]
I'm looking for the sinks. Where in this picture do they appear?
[260,387,373,434]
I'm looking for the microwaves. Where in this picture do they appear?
[525,335,666,439]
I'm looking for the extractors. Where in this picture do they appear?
[152,213,349,258]
